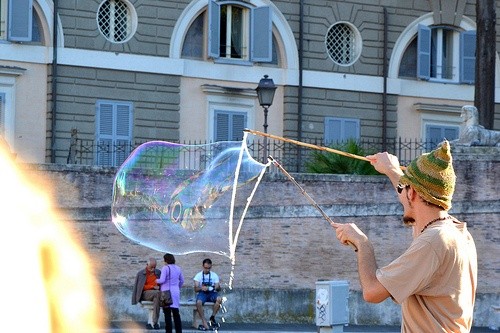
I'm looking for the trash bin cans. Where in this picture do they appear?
[315,280,349,333]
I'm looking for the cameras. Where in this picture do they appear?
[206,285,214,291]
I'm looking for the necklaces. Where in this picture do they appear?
[421,216,451,232]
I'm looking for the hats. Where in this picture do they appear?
[400,140,457,210]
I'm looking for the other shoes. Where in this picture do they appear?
[210,316,216,325]
[198,325,207,331]
[154,324,159,329]
[147,324,153,329]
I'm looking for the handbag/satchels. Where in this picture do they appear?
[156,290,172,307]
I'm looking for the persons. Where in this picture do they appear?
[193,258,222,330]
[331,138,478,333]
[132,258,162,329]
[155,253,185,333]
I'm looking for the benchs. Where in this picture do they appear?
[140,299,216,326]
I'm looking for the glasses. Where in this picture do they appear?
[396,183,409,194]
[204,266,211,269]
